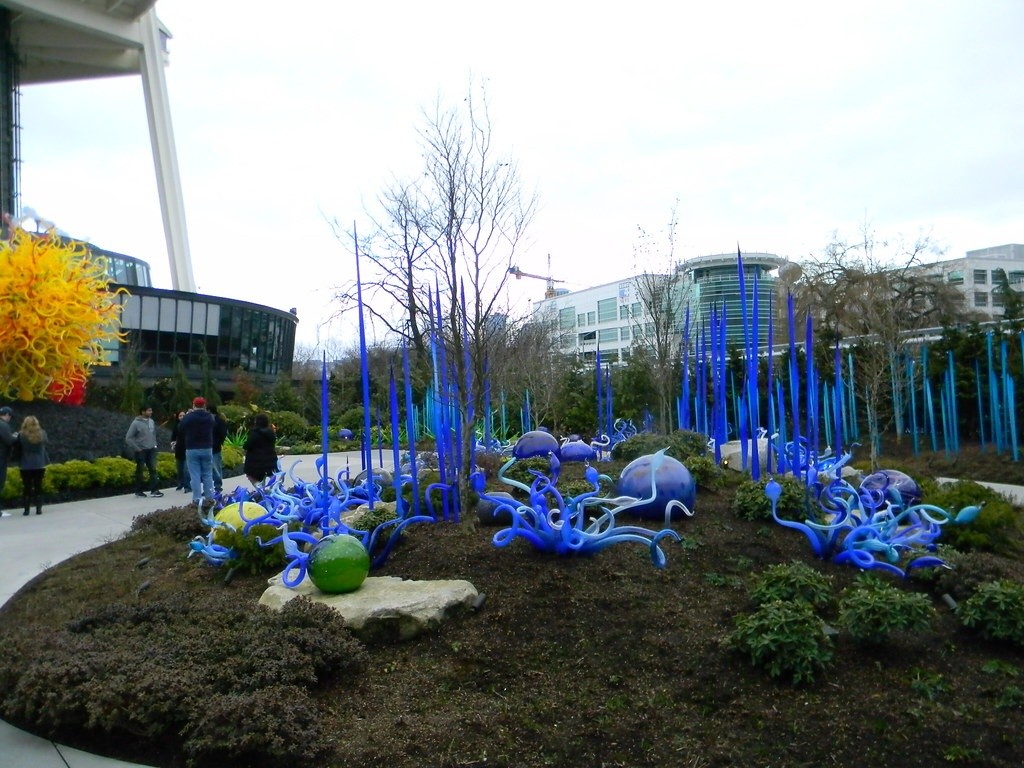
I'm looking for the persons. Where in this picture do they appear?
[125,404,164,498]
[177,396,214,503]
[13,415,50,516]
[0,407,20,518]
[200,403,227,500]
[245,413,277,488]
[171,410,193,495]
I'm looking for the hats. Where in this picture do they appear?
[192,397,205,405]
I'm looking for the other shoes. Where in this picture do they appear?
[1,513,12,517]
[176,486,191,494]
[21,509,41,516]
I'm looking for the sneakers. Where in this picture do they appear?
[136,492,163,497]
